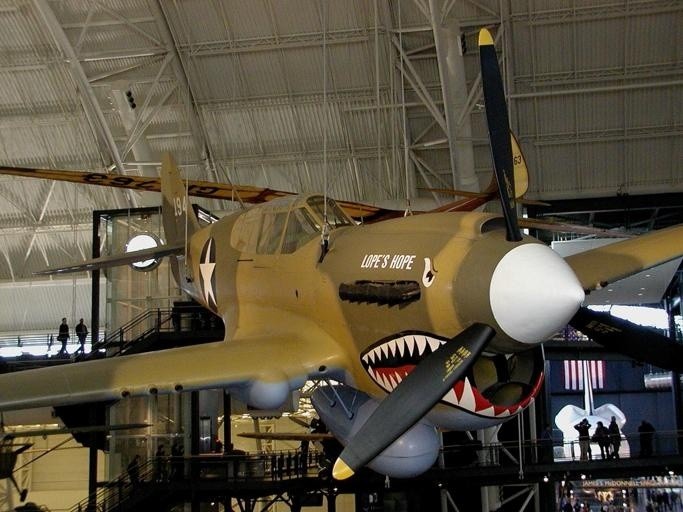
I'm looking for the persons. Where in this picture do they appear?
[556,485,683,511]
[540,424,553,463]
[126,453,140,490]
[58,317,67,353]
[573,420,591,460]
[606,415,622,459]
[592,420,610,459]
[211,435,222,453]
[73,318,89,354]
[170,438,180,457]
[151,444,167,483]
[637,419,656,457]
[582,418,592,460]
[174,444,185,476]
